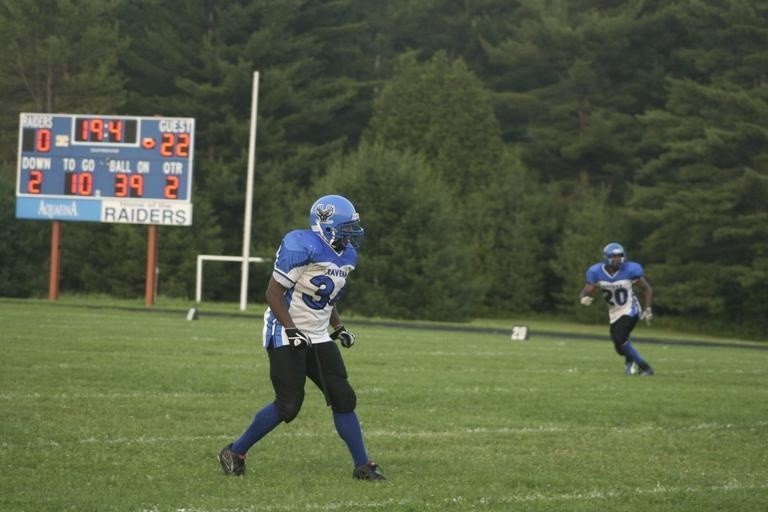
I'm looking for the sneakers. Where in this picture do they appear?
[640,369,655,376]
[217,444,246,476]
[624,361,636,375]
[353,464,384,480]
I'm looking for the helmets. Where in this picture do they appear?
[310,195,362,250]
[604,243,624,270]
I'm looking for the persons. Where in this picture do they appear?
[214,192,389,484]
[578,242,656,378]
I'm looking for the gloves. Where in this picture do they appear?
[640,307,652,327]
[286,328,312,349]
[331,328,355,347]
[580,296,594,306]
